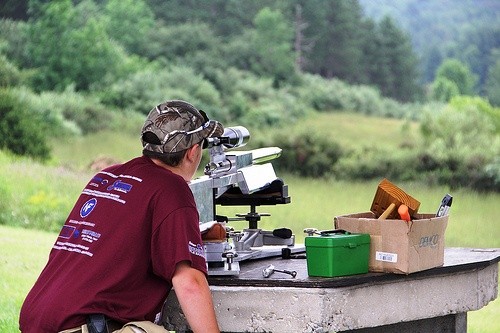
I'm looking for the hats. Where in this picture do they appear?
[140,100,224,154]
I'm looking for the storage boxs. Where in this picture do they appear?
[334,212,449,275]
[305,233,371,277]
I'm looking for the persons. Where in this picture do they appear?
[19,100,224,333]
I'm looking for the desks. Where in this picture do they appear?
[157,247,500,333]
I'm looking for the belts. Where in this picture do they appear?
[70,319,125,333]
